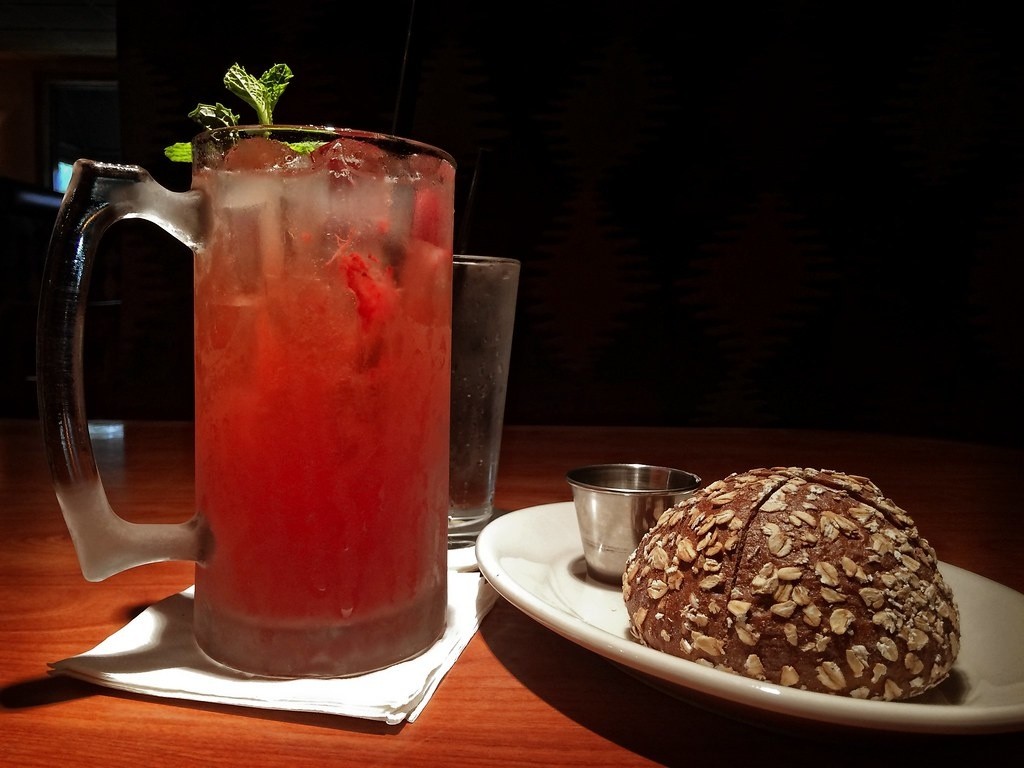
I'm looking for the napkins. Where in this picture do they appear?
[50,543,493,729]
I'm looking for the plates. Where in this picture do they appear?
[474,500,1024,736]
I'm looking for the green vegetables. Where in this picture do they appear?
[164,61,328,162]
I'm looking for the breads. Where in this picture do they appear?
[620,465,960,701]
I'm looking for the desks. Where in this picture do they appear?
[0,418,1024,768]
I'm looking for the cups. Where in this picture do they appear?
[443,252,522,552]
[25,123,450,681]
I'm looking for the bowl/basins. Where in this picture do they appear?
[564,462,702,588]
